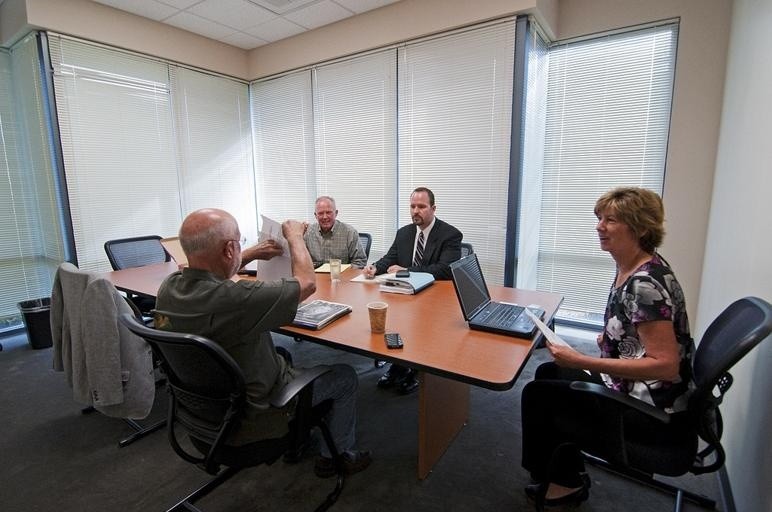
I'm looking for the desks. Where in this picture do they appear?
[91,260,566,484]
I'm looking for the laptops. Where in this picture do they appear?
[448,253,545,340]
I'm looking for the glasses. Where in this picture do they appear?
[229,236,247,246]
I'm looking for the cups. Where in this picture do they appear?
[367,301,389,333]
[329,259,342,282]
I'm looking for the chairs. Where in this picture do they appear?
[58,262,169,449]
[535,295,772,512]
[120,313,345,512]
[293,232,372,345]
[103,235,171,313]
[374,241,475,370]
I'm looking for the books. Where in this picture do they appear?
[379,271,435,296]
[293,299,353,330]
[315,263,351,273]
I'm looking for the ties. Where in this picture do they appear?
[413,231,425,268]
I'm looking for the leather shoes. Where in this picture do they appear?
[313,449,374,479]
[377,366,412,389]
[524,472,592,507]
[393,374,419,396]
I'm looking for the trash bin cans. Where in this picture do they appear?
[16,297,53,350]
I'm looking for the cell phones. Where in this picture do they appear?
[384,333,403,349]
[395,270,410,278]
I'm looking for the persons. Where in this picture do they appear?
[362,187,463,395]
[302,195,367,270]
[153,207,373,481]
[520,186,696,512]
